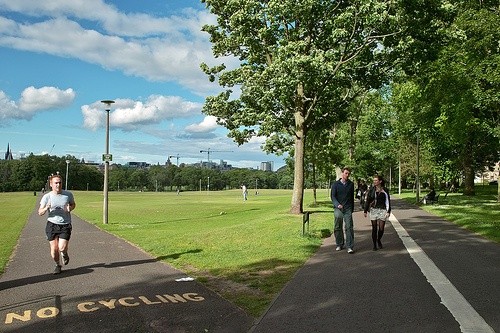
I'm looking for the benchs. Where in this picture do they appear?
[424,195,439,203]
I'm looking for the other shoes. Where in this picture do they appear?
[378,242,382,248]
[372,243,376,251]
[347,248,354,253]
[63,255,69,266]
[336,246,341,250]
[53,265,61,274]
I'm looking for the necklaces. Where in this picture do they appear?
[376,185,383,190]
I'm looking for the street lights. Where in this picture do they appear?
[56,171,60,176]
[100,100,115,225]
[65,160,71,191]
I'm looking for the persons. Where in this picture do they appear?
[363,175,391,251]
[419,187,435,203]
[176,189,179,196]
[240,185,247,201]
[355,180,373,209]
[331,168,355,253]
[38,175,75,274]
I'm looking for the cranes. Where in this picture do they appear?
[169,153,199,167]
[200,148,234,162]
